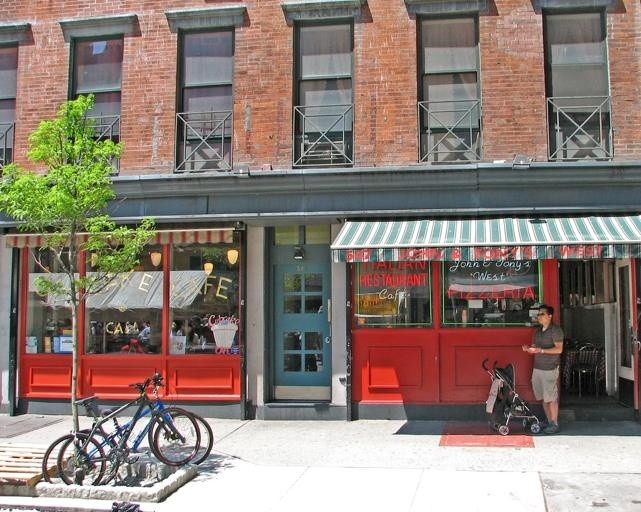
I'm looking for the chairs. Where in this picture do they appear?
[560,337,605,401]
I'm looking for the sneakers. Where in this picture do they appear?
[540,421,560,434]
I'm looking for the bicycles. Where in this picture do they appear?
[42,373,213,486]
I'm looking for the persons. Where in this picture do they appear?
[126,320,159,353]
[46,317,56,342]
[521,305,565,434]
[186,315,216,343]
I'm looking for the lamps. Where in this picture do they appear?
[512,152,531,171]
[233,164,251,179]
[203,261,214,276]
[226,249,239,265]
[150,252,162,267]
[292,246,305,260]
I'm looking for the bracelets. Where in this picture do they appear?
[540,348,544,355]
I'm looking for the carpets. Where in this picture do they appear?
[438,422,535,449]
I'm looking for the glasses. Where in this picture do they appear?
[537,313,546,317]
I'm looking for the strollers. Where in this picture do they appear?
[482,358,542,436]
